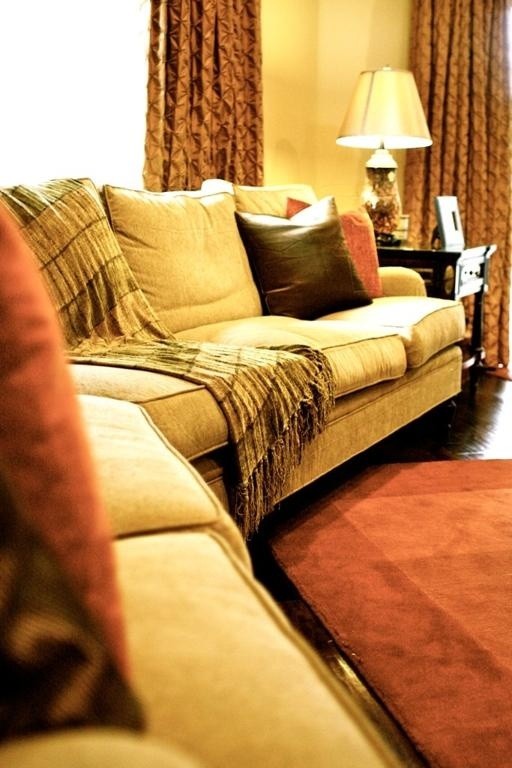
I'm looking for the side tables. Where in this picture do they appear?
[378,244,500,390]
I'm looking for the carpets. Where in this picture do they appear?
[271,453,510,766]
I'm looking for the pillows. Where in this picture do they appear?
[3,177,148,350]
[232,183,317,218]
[1,205,151,737]
[233,197,373,319]
[102,181,262,333]
[287,194,380,301]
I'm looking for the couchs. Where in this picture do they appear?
[71,265,466,732]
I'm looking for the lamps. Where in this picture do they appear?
[337,65,432,245]
